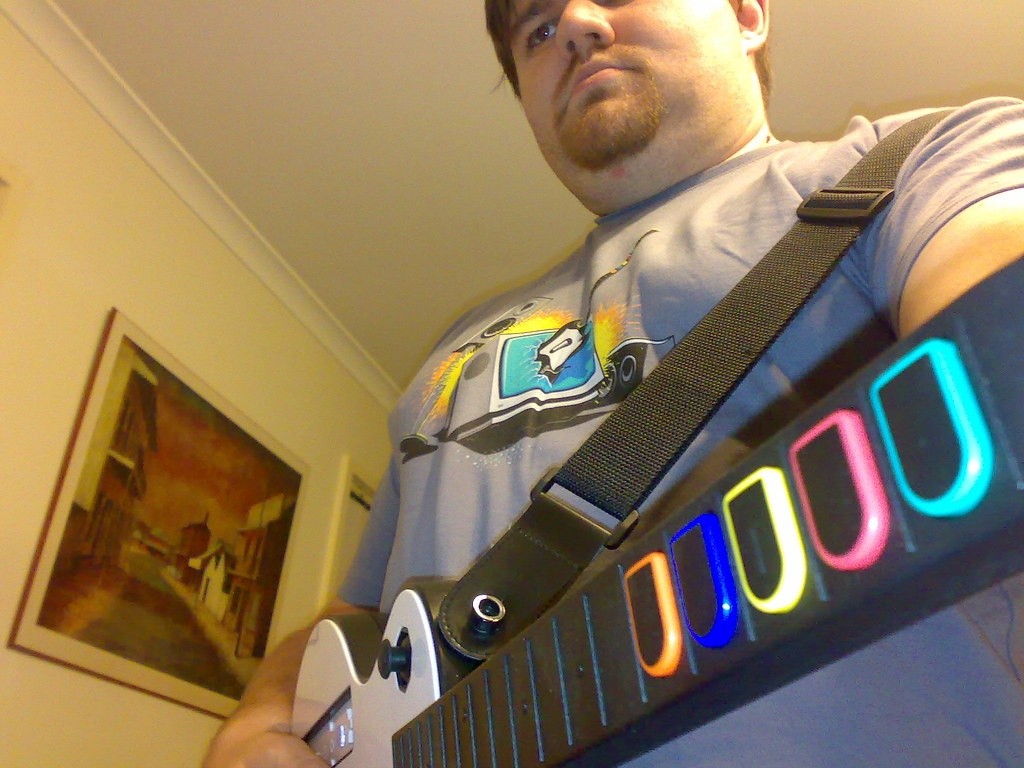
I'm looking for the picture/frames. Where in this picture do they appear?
[6,307,310,717]
[316,455,382,616]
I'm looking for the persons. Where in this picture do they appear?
[200,0,1024,768]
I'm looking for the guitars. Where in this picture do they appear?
[288,250,1024,768]
